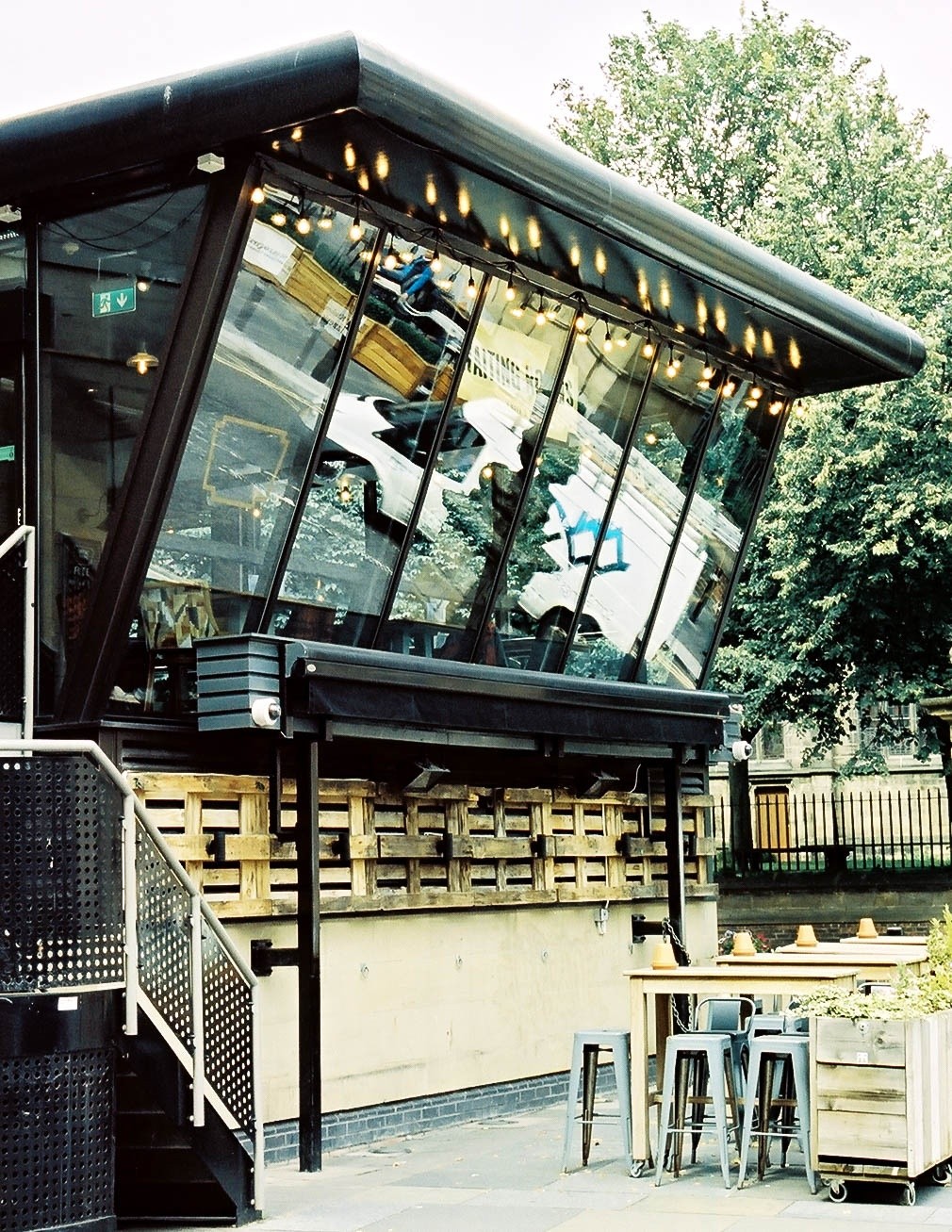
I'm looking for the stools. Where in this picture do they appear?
[566,997,815,1194]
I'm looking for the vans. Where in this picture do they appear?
[291,388,712,667]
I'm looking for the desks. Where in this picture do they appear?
[626,967,860,1170]
[778,941,931,956]
[841,936,929,944]
[715,954,928,1034]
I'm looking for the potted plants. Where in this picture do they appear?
[791,904,952,1204]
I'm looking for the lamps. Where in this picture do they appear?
[569,768,621,798]
[127,341,159,374]
[390,759,450,794]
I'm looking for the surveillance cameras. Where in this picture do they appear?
[733,740,753,761]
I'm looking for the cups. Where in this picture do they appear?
[796,925,818,946]
[733,932,756,956]
[858,917,878,938]
[651,943,679,970]
